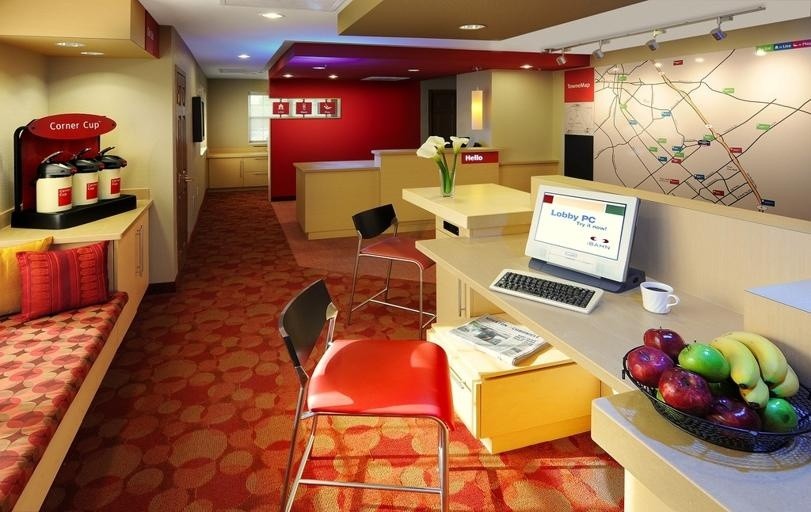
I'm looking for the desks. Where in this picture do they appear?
[414,177,811,510]
[1,197,156,320]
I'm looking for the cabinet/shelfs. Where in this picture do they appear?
[242,148,268,188]
[207,150,243,191]
[399,181,532,322]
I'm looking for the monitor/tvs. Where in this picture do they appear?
[524,184,646,295]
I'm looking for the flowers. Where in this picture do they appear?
[416,136,469,193]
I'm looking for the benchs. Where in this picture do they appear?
[0,293,127,512]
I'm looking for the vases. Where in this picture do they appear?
[439,165,460,196]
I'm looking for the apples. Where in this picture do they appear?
[659,367,715,414]
[703,397,761,431]
[763,397,796,432]
[707,381,732,398]
[678,342,730,382]
[626,346,676,389]
[654,386,665,403]
[643,325,685,364]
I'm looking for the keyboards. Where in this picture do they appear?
[488,268,604,315]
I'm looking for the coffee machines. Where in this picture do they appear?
[35,150,78,213]
[97,147,127,200]
[69,148,99,206]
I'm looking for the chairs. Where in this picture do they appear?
[277,278,454,511]
[348,201,435,340]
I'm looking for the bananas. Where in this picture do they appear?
[710,330,800,409]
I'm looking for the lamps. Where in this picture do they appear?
[645,31,664,50]
[711,19,727,41]
[470,66,483,129]
[555,50,571,70]
[593,41,609,61]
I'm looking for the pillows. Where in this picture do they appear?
[1,236,53,316]
[14,242,109,324]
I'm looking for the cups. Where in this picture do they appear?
[639,282,679,316]
